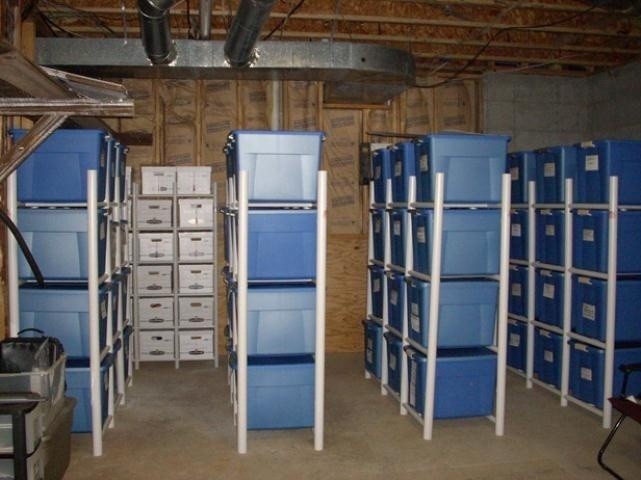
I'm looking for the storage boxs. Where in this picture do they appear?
[0,350,79,480]
[225,125,327,202]
[10,129,128,201]
[139,164,213,196]
[368,129,639,202]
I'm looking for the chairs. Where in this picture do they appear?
[597,361,639,479]
